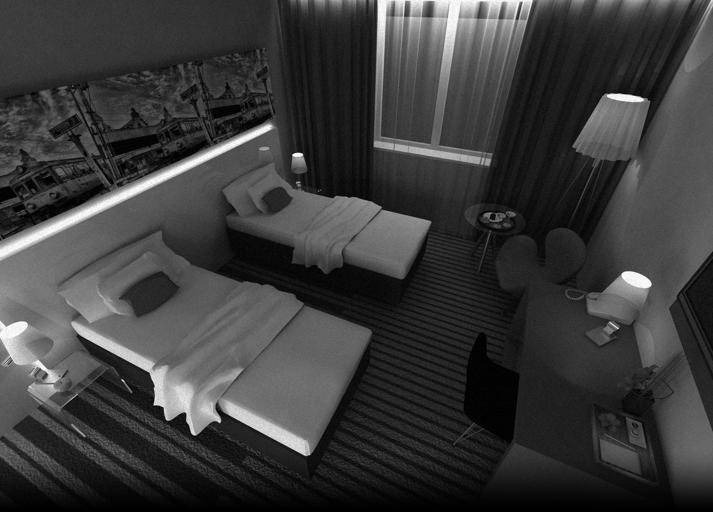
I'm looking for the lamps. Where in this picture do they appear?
[291,152,307,192]
[585,270,651,348]
[567,93,651,229]
[0,320,68,384]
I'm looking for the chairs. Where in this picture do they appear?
[451,331,519,447]
[494,227,588,314]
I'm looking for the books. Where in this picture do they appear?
[599,437,643,478]
[595,406,647,451]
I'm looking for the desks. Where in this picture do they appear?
[471,279,676,512]
[464,202,525,275]
[26,349,133,438]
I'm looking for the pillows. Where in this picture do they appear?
[54,230,190,324]
[221,162,294,218]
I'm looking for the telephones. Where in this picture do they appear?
[586,292,640,326]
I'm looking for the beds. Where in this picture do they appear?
[224,190,433,306]
[70,263,374,481]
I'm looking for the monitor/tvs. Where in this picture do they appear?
[677,252,713,377]
